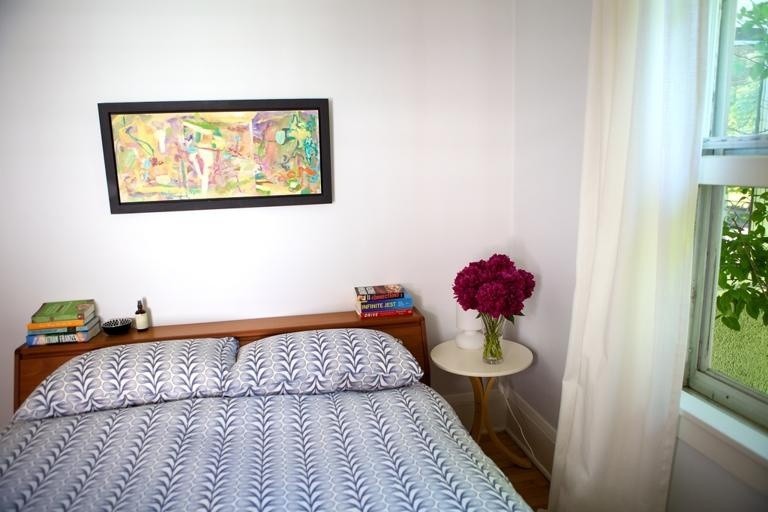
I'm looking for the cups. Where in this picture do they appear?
[456,298,486,350]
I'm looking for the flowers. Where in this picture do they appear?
[451,253,535,359]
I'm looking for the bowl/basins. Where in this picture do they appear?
[101,317,133,336]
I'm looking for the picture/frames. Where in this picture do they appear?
[97,98,332,214]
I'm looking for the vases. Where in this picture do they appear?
[480,315,505,364]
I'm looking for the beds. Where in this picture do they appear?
[0,308,531,512]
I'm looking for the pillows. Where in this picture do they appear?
[224,327,426,398]
[10,337,239,420]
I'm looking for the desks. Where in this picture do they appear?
[429,337,534,469]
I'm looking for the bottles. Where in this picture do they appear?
[134,300,149,333]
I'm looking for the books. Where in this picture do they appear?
[352,285,405,302]
[25,321,103,346]
[353,304,413,318]
[27,310,97,331]
[353,288,413,313]
[31,299,97,323]
[27,315,100,334]
